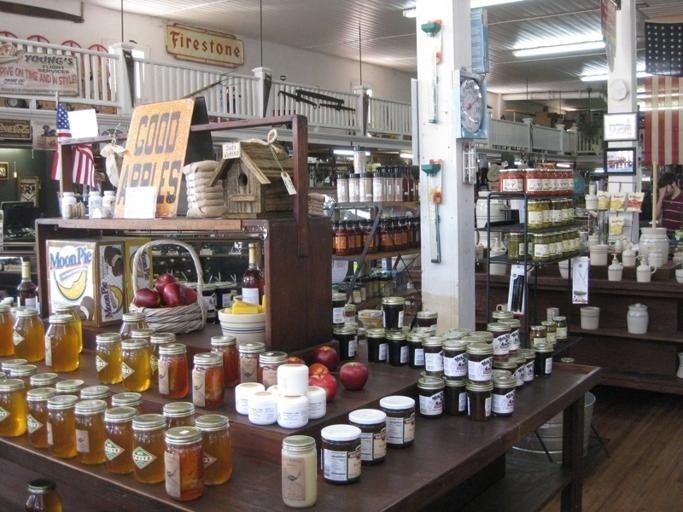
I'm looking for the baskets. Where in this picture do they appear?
[126,240,209,338]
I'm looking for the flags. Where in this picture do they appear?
[51,91,95,188]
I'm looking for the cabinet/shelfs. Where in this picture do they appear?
[1,117,602,511]
[485,191,609,465]
[313,182,421,309]
[468,261,682,399]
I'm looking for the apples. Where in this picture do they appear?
[309,374,337,401]
[339,361,369,390]
[309,363,329,376]
[314,346,338,369]
[288,356,305,364]
[163,283,183,306]
[134,288,161,307]
[154,274,176,293]
[182,288,197,304]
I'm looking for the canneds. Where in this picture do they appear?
[498,168,580,260]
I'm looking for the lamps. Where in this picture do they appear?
[512,40,605,57]
[402,9,416,18]
[580,71,653,82]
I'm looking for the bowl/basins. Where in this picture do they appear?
[217,308,264,344]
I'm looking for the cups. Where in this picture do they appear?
[579,306,599,330]
[589,244,607,266]
[546,308,559,322]
[675,269,683,284]
[495,303,507,311]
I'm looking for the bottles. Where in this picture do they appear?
[277,396,308,428]
[388,332,409,365]
[381,218,393,251]
[122,339,151,391]
[497,318,520,351]
[407,335,429,367]
[360,172,372,203]
[80,386,112,408]
[234,382,265,414]
[74,400,108,464]
[45,315,80,372]
[468,343,493,381]
[488,323,510,356]
[239,342,265,383]
[348,173,360,203]
[360,219,367,233]
[512,349,535,382]
[493,375,515,415]
[193,352,225,409]
[16,256,36,307]
[0,378,27,437]
[0,359,85,395]
[111,392,144,413]
[307,386,326,420]
[331,221,338,253]
[407,217,415,248]
[334,329,356,358]
[384,174,395,201]
[120,313,149,340]
[0,304,82,362]
[422,338,443,373]
[194,414,233,486]
[530,326,546,347]
[336,174,348,202]
[96,333,122,385]
[162,401,196,429]
[348,408,386,465]
[399,217,408,250]
[373,173,384,201]
[256,350,288,388]
[392,218,401,250]
[47,394,78,458]
[211,336,239,387]
[104,406,137,474]
[415,311,438,331]
[552,316,568,339]
[507,357,524,389]
[382,297,405,330]
[132,413,168,484]
[62,192,77,218]
[366,329,386,361]
[25,478,65,512]
[468,381,491,420]
[102,191,115,208]
[277,364,309,396]
[444,341,468,377]
[380,395,415,448]
[364,219,376,254]
[164,427,205,502]
[492,310,514,322]
[414,216,420,247]
[242,242,264,305]
[493,361,517,375]
[345,220,355,254]
[339,274,393,303]
[394,174,403,201]
[535,349,553,375]
[157,343,189,398]
[398,167,409,202]
[281,435,318,508]
[26,387,56,449]
[353,220,362,255]
[88,191,102,219]
[418,376,444,418]
[336,221,348,256]
[131,330,153,341]
[149,333,177,384]
[248,392,277,425]
[320,424,362,485]
[405,167,415,201]
[446,378,466,415]
[541,321,557,344]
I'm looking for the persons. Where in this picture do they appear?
[656,171,683,235]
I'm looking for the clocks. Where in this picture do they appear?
[456,66,492,141]
